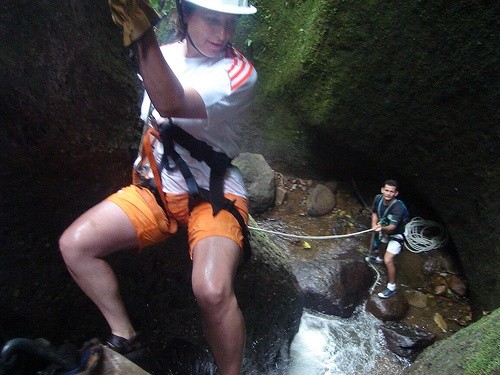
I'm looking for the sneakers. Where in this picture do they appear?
[365,256,382,265]
[377,287,397,299]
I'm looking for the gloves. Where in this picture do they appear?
[109,0,162,47]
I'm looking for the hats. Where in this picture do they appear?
[183,0,257,15]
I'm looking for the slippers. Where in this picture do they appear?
[103,333,141,355]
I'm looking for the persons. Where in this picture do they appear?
[59,0,258,375]
[371,180,408,298]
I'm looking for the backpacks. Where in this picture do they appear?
[377,195,409,226]
